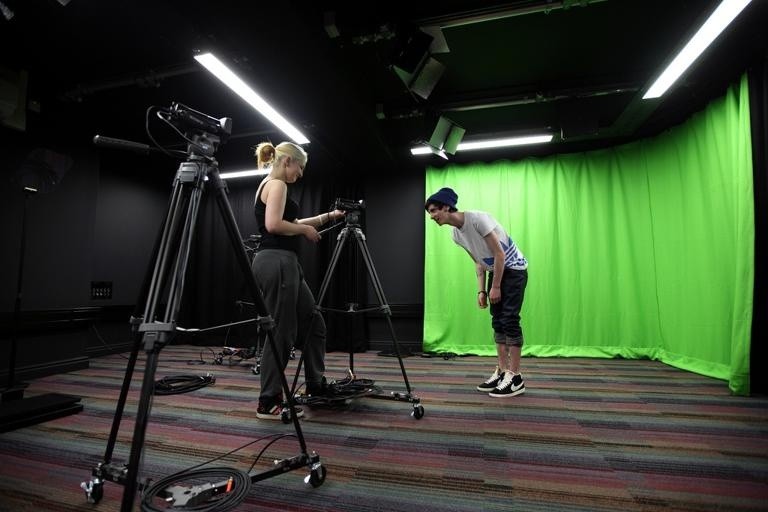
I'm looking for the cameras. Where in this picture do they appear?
[332,195,363,215]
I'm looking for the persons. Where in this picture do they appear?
[249,139,346,421]
[424,187,528,398]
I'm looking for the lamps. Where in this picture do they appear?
[424,115,467,162]
[389,54,446,105]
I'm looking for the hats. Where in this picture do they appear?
[427,188,458,211]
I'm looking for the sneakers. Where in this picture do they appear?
[476,366,525,398]
[256,395,304,420]
[302,376,345,405]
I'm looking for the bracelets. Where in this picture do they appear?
[328,211,330,221]
[478,290,487,295]
[318,214,323,226]
